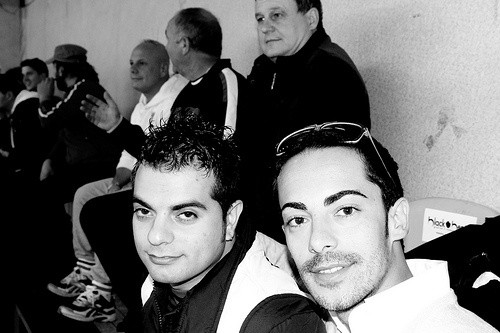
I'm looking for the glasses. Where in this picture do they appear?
[275,122,395,185]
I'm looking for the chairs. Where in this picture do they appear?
[403,197,499,254]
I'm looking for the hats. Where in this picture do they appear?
[44,44,87,66]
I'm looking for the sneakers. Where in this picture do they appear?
[58,284,116,322]
[48,266,91,297]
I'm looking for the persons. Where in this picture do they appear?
[245,0,370,246]
[80,8,272,333]
[132,108,327,333]
[271,122,500,332]
[47,39,189,322]
[0,44,116,281]
